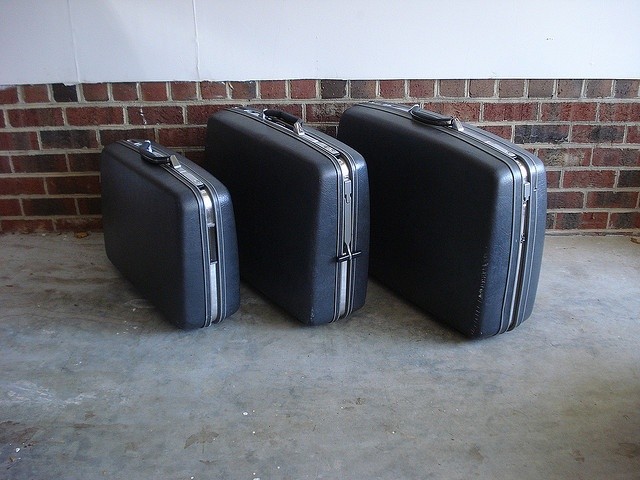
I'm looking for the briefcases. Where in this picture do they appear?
[204,105,371,326]
[100,138,241,331]
[339,101,547,340]
